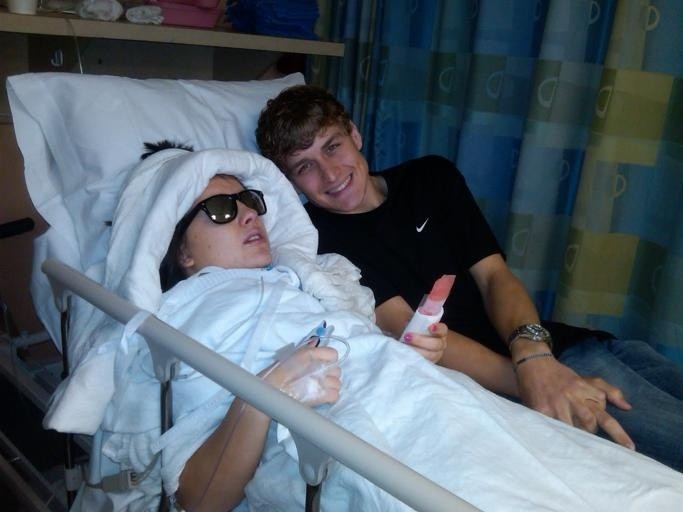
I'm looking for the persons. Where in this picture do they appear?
[252,84,683,475]
[118,139,683,512]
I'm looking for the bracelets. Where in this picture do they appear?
[515,352,552,370]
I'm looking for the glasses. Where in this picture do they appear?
[178,189,268,239]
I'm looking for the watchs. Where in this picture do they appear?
[506,323,555,348]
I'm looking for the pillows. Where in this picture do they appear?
[4,68,308,274]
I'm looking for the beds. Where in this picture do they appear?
[3,226,683,512]
